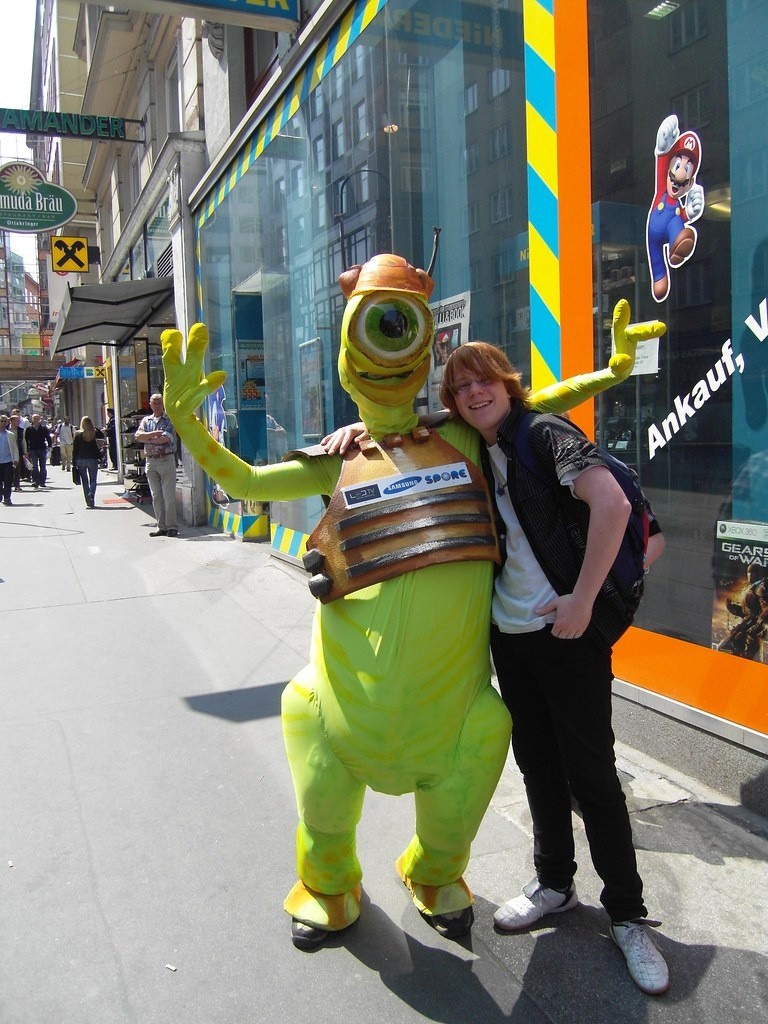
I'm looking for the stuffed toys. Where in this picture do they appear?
[159,253,666,949]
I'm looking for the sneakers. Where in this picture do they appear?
[609,917,671,995]
[493,878,578,930]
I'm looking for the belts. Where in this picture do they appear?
[148,451,173,457]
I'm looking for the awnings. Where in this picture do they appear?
[51,276,174,359]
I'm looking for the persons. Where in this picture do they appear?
[323,339,670,995]
[0,407,76,504]
[71,416,105,508]
[101,409,118,471]
[134,394,180,537]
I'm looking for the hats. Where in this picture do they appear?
[107,408,114,414]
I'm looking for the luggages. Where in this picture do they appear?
[50,443,61,466]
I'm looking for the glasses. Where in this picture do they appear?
[452,376,496,397]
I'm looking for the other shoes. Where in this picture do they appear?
[17,488,23,493]
[62,466,65,470]
[39,483,46,487]
[32,482,38,487]
[87,493,94,508]
[110,467,118,471]
[166,529,178,537]
[149,530,168,537]
[67,469,70,471]
[3,500,12,505]
[15,487,19,492]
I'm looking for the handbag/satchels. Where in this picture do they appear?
[515,411,650,598]
[72,465,81,485]
